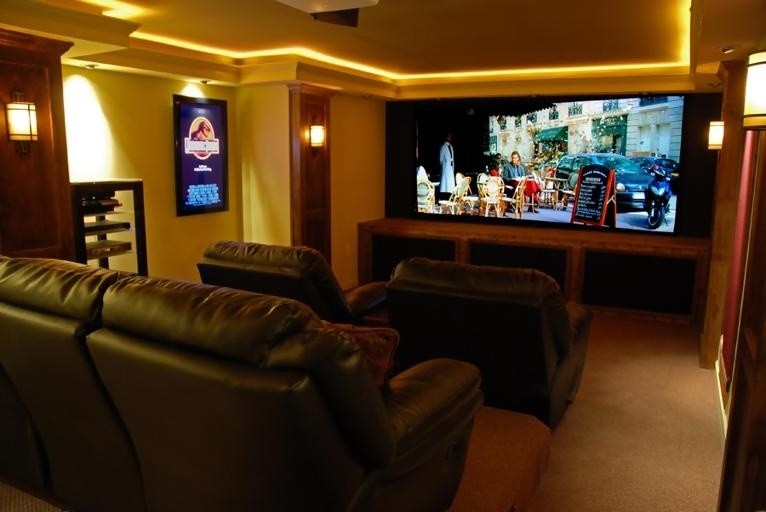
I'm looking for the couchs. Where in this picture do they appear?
[0,251,555,512]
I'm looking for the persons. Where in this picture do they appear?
[416,130,539,216]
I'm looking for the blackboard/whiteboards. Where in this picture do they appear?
[571,164,610,224]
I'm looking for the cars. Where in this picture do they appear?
[628,156,679,185]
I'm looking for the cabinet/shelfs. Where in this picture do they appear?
[67,177,151,278]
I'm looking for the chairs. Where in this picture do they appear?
[385,253,600,430]
[196,238,395,337]
[417,166,579,219]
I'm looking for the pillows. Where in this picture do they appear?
[316,315,404,402]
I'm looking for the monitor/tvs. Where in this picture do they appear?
[384,92,725,241]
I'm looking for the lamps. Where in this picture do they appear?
[4,89,40,160]
[739,49,766,133]
[307,112,327,158]
[706,119,729,153]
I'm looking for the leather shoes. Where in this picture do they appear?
[528,208,538,213]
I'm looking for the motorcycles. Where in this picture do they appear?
[642,166,674,229]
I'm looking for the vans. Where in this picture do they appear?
[553,152,672,211]
